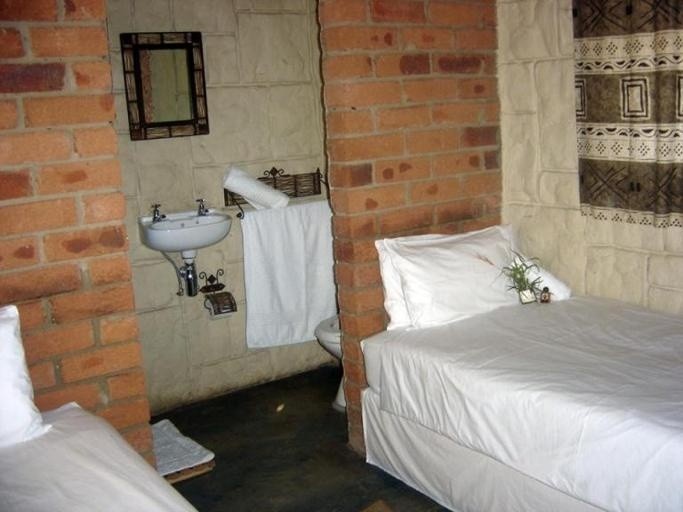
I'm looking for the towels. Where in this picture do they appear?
[240,199,338,350]
[221,168,289,212]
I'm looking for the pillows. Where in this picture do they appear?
[387,223,572,328]
[0,304,51,449]
[373,234,453,310]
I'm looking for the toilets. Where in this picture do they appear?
[314,315,346,414]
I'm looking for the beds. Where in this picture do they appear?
[0,403,203,512]
[357,295,680,512]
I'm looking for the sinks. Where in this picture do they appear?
[137,208,232,259]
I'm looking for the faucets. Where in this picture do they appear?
[151,204,166,223]
[195,198,210,215]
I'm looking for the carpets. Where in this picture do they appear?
[164,454,217,484]
[152,417,216,476]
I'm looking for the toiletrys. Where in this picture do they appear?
[539,287,550,303]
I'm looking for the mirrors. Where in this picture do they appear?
[117,31,210,141]
